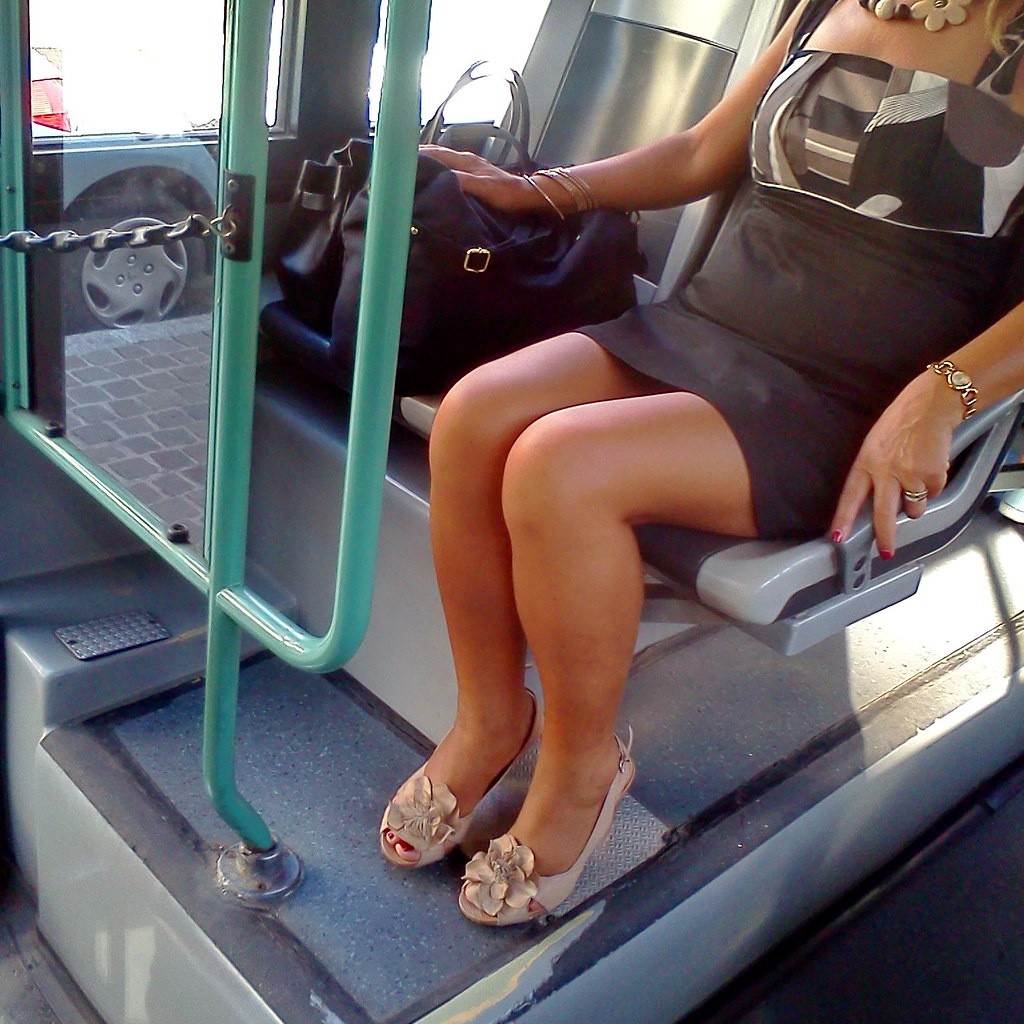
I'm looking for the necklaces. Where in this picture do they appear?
[858,1,971,35]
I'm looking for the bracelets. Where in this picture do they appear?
[524,165,599,222]
[926,360,978,421]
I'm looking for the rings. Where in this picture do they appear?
[902,488,930,503]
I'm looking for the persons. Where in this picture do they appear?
[381,0,1023,929]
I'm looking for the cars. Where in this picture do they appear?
[30,49,77,137]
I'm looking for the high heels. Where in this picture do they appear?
[459,726,636,926]
[380,686,540,869]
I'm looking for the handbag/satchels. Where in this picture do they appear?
[329,122,647,396]
[275,59,531,333]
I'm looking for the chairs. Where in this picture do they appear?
[259,0,796,439]
[634,169,1023,654]
